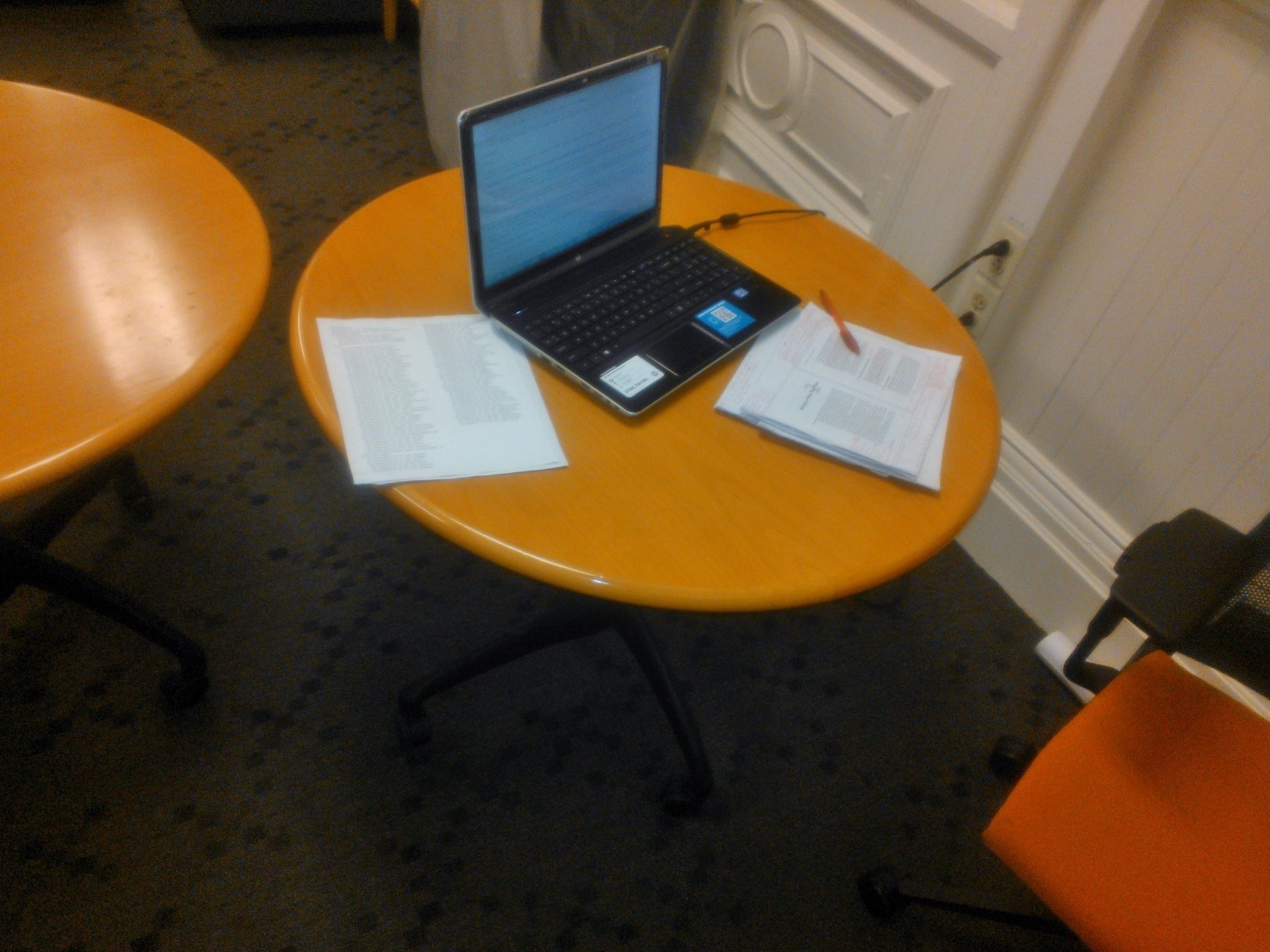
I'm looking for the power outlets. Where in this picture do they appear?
[961,219,1028,337]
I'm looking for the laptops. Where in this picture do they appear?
[459,48,801,415]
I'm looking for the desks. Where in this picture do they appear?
[1,79,270,675]
[288,164,1002,818]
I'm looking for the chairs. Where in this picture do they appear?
[984,514,1270,952]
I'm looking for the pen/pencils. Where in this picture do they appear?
[818,288,860,355]
